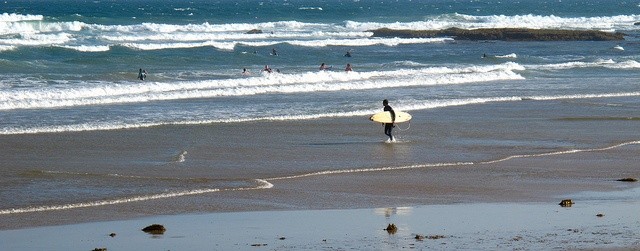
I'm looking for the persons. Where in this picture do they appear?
[321,63,326,71]
[242,68,249,75]
[138,69,147,78]
[345,63,352,71]
[382,99,395,140]
[268,69,273,73]
[137,70,146,80]
[263,65,270,71]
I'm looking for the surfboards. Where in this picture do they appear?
[369,111,412,123]
